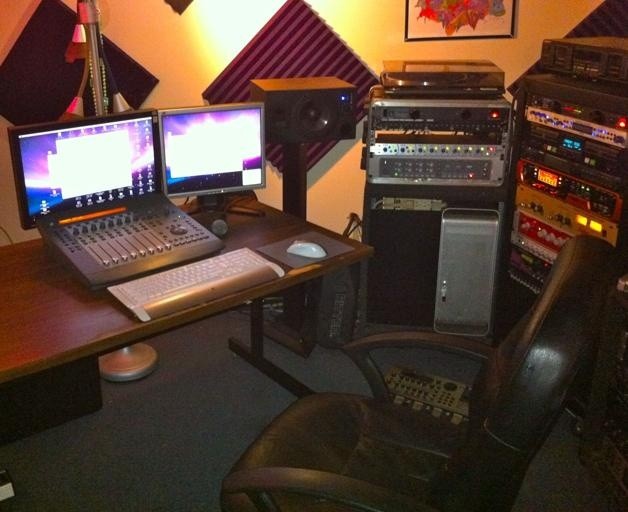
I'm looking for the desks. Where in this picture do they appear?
[1,205,374,447]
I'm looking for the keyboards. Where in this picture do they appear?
[107,247,285,322]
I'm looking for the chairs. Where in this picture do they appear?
[216,232,622,509]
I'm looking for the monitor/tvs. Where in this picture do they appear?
[157,102,266,217]
[7,108,161,230]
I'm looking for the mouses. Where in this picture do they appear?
[287,240,327,259]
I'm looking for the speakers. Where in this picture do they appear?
[249,74,356,144]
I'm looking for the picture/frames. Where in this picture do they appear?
[403,0,519,43]
